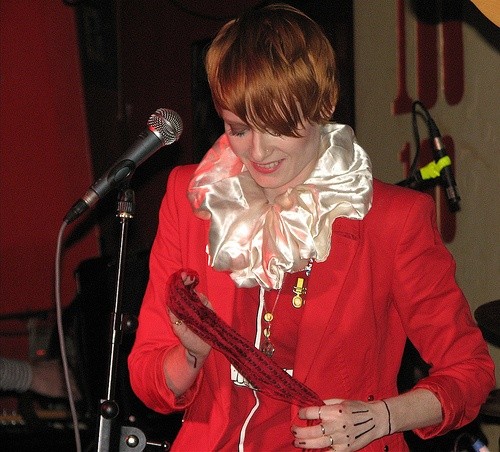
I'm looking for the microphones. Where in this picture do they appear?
[428,118,460,207]
[63,108,183,225]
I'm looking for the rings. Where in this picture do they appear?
[173,321,182,326]
[329,435,334,447]
[320,424,325,437]
[318,407,321,420]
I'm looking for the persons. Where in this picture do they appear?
[127,2,496,452]
[0,358,84,402]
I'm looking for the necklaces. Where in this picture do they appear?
[260,273,289,357]
